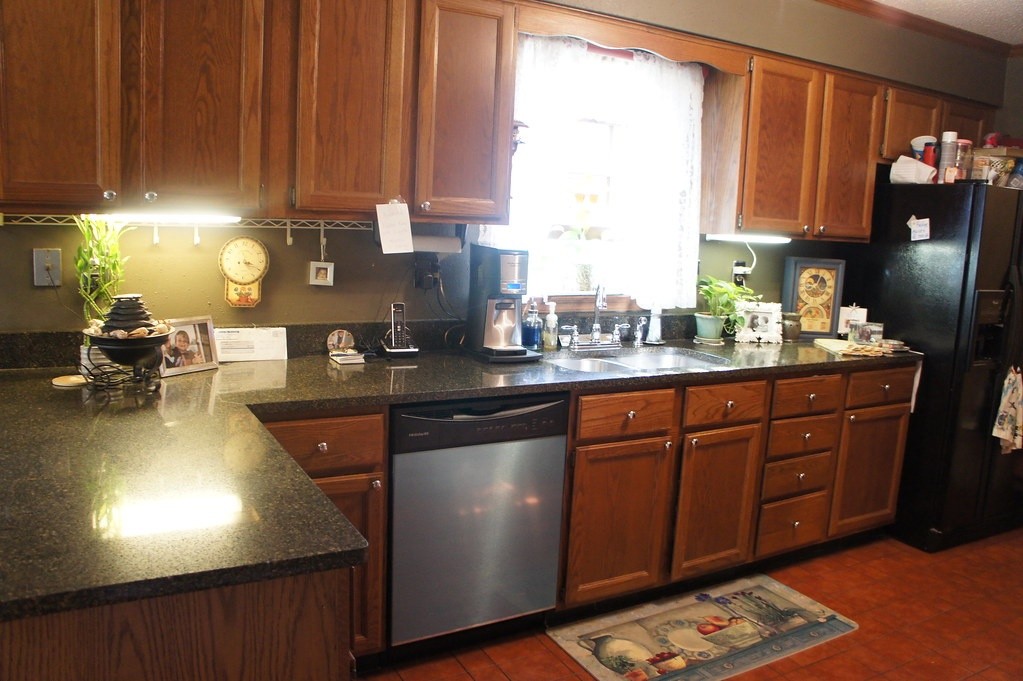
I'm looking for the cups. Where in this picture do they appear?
[880,339,904,349]
[910,134,934,159]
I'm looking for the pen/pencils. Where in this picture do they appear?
[330,353,376,357]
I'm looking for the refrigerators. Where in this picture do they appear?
[873,163,1023,552]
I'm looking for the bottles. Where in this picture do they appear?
[924,131,975,183]
[545,301,560,349]
[522,294,543,352]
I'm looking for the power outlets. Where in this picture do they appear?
[416,254,439,290]
[32,248,62,287]
[734,261,749,286]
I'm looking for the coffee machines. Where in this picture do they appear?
[460,240,543,362]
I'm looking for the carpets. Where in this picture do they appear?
[542,570,855,681]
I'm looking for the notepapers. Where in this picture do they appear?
[329,351,365,364]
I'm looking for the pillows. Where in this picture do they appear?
[882,86,993,166]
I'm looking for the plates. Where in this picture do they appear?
[887,347,911,352]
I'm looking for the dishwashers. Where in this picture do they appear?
[383,391,571,649]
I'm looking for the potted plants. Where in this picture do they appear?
[686,276,758,344]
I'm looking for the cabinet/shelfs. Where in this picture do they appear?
[0,0,264,219]
[260,414,394,653]
[567,381,771,604]
[295,0,515,220]
[831,371,920,533]
[702,54,880,235]
[755,375,850,554]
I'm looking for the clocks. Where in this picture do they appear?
[218,237,270,307]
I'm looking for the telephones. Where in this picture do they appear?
[379,302,419,358]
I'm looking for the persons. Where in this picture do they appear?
[744,313,768,332]
[159,331,203,367]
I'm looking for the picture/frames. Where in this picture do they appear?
[779,256,845,338]
[733,298,785,344]
[309,260,335,286]
[155,315,220,377]
